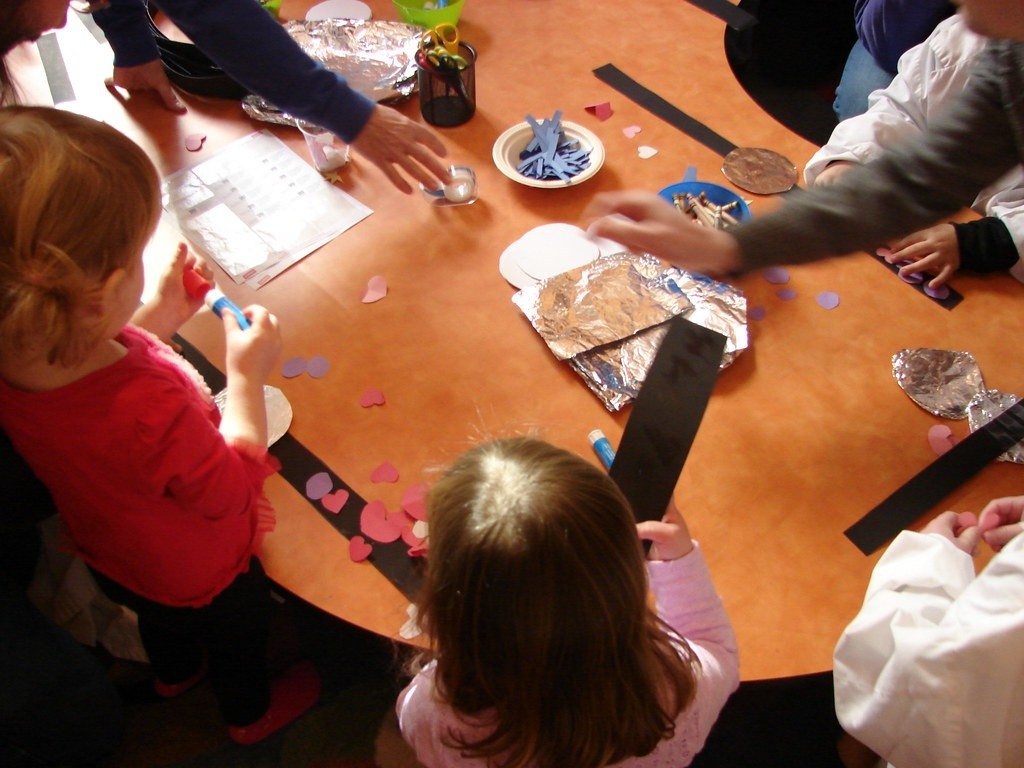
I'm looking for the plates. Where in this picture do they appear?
[492,118,606,189]
[305,0,372,22]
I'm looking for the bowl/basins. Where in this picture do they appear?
[392,0,464,35]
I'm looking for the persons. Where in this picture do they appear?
[1,106,325,747]
[588,0,1024,294]
[89,0,454,195]
[834,497,1024,768]
[397,434,739,768]
[0,0,71,98]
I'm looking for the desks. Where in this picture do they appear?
[57,0,1024,687]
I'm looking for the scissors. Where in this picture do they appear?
[418,23,469,107]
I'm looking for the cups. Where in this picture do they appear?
[414,40,478,127]
[296,119,352,172]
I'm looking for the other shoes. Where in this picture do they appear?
[154,646,209,697]
[228,660,320,745]
